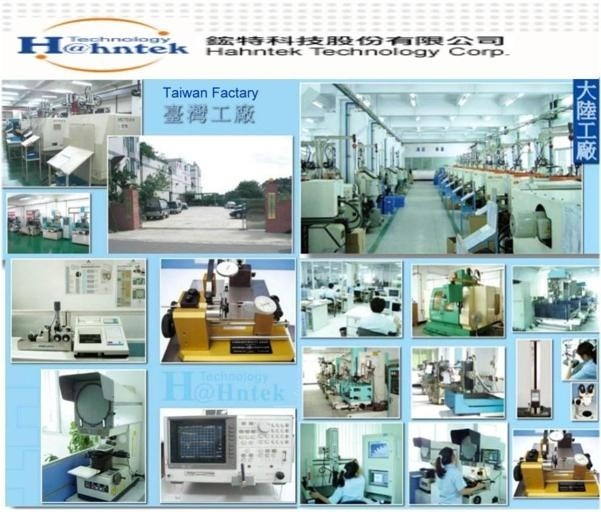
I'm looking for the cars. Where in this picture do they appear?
[145,195,191,221]
[224,200,249,221]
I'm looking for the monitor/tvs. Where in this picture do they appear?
[367,440,390,459]
[383,300,393,312]
[387,289,400,298]
[369,469,388,487]
[165,415,236,470]
[481,448,501,469]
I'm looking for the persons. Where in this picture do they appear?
[355,283,363,301]
[354,297,402,336]
[564,341,597,380]
[308,462,366,504]
[434,446,487,505]
[325,283,342,314]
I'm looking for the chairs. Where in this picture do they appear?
[356,326,386,335]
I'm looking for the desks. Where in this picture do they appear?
[302,287,373,332]
[346,303,402,338]
